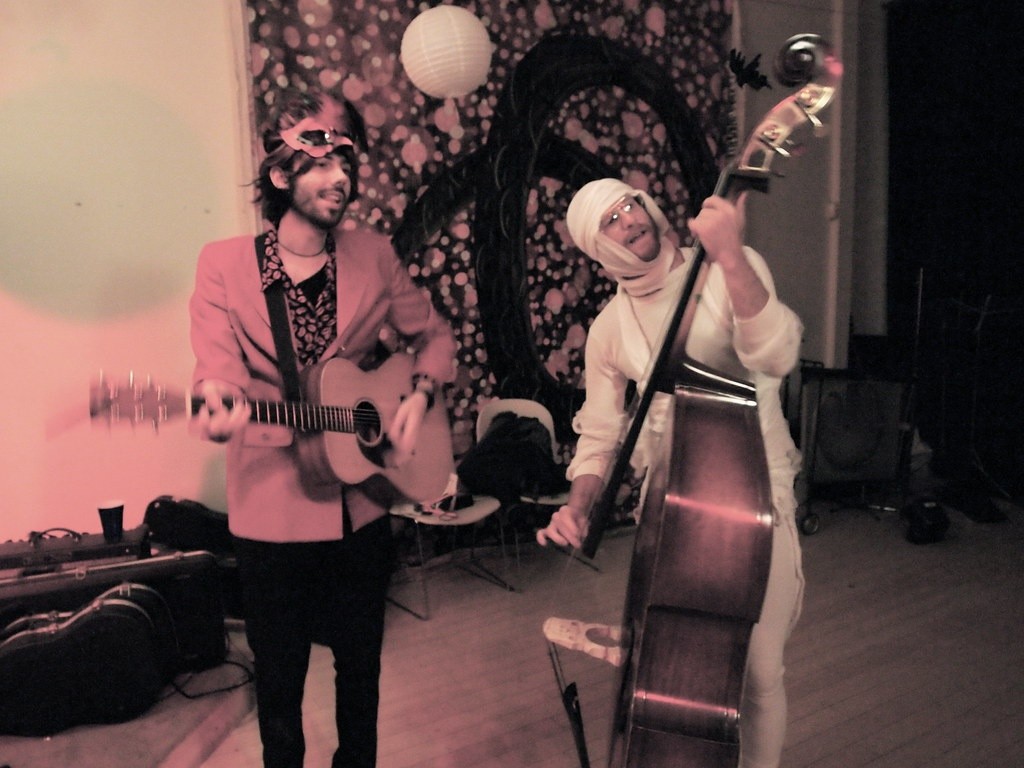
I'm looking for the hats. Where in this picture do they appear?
[566,178,635,261]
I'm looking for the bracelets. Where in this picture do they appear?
[416,387,436,412]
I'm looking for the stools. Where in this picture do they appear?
[385,492,515,622]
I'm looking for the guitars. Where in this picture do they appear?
[90,354,453,506]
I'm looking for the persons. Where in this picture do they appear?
[187,118,459,768]
[536,178,807,768]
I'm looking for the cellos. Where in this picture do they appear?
[543,34,843,768]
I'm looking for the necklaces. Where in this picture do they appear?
[278,240,325,257]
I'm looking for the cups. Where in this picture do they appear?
[98,501,124,540]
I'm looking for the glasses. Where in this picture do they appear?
[600,194,634,230]
[295,129,356,146]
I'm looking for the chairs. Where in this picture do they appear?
[469,398,600,593]
